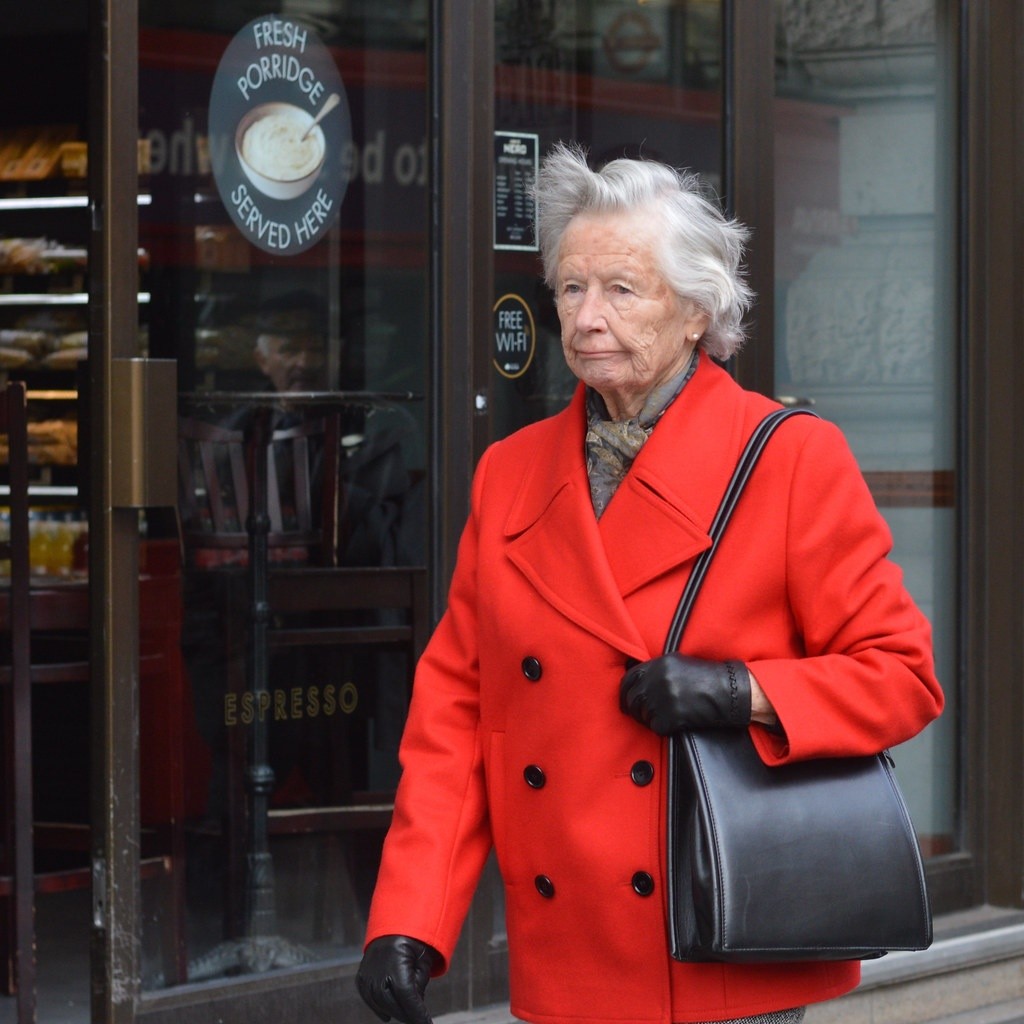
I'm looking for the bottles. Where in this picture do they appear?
[1,509,89,581]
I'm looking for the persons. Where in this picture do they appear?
[353,142,947,1024]
[183,281,425,567]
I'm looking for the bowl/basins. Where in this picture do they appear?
[236,102,326,200]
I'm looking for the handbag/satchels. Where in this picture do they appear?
[667,734,934,964]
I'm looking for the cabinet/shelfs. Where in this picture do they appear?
[0,75,381,569]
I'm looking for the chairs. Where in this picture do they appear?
[0,380,432,1023]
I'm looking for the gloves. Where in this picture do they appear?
[357,934,440,1024]
[618,655,754,736]
